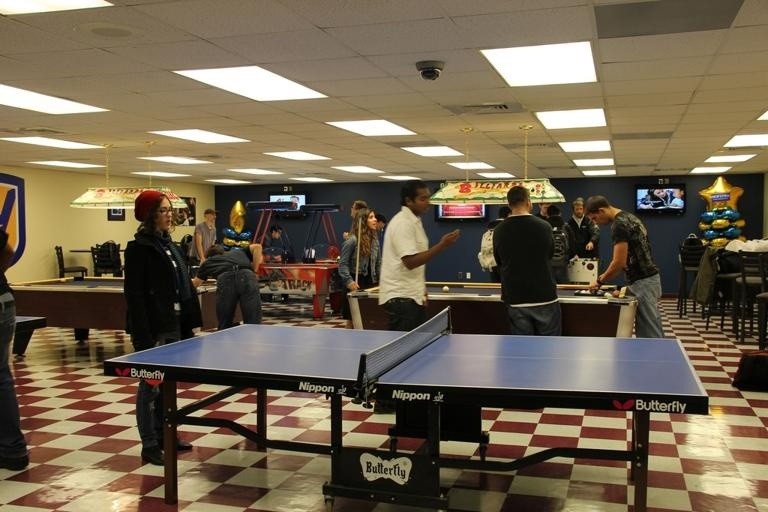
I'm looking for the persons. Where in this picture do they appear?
[583,195,664,338]
[337,199,387,329]
[488,207,512,282]
[535,197,601,284]
[492,186,562,336]
[671,188,684,209]
[379,180,461,332]
[256,225,282,248]
[191,243,263,330]
[0,230,30,471]
[639,189,675,209]
[124,191,203,466]
[189,208,217,278]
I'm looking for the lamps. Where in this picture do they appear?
[65,140,193,213]
[425,125,565,207]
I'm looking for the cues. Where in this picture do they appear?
[356,218,362,285]
[464,285,618,289]
[202,279,274,284]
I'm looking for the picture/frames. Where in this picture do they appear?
[107,209,126,221]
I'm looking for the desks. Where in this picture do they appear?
[9,279,242,346]
[347,281,638,338]
[245,263,347,321]
[104,324,709,512]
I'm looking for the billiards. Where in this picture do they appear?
[443,286,449,292]
[574,288,619,297]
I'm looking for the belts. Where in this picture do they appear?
[215,265,256,279]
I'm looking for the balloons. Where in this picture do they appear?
[697,175,746,247]
[223,200,253,252]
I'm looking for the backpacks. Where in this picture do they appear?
[547,222,569,269]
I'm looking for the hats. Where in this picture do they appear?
[205,209,220,214]
[133,189,166,222]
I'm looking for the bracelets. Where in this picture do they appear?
[597,278,604,285]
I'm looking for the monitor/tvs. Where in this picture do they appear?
[434,188,488,222]
[267,192,311,219]
[633,184,687,216]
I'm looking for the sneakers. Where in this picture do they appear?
[0,455,30,471]
[162,438,194,452]
[140,445,167,466]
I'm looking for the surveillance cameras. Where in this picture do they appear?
[416,61,444,81]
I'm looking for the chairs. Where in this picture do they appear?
[679,237,768,352]
[53,239,126,280]
